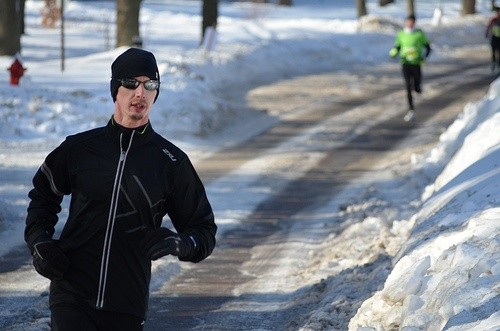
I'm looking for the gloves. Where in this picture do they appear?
[137,227,193,261]
[30,234,72,281]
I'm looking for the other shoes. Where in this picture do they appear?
[404,110,414,121]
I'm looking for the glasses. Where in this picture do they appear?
[118,78,160,91]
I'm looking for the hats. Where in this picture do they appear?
[110,48,160,104]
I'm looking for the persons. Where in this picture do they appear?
[388,15,432,122]
[484,6,500,70]
[24,48,217,331]
